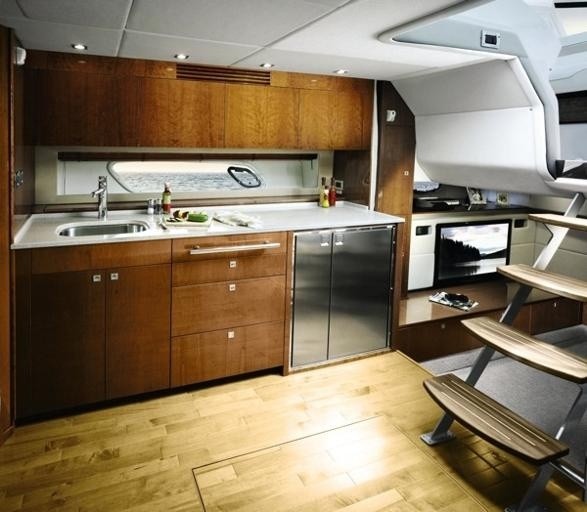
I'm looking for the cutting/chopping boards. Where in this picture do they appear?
[162,213,212,227]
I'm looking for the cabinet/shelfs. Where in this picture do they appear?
[24,71,225,152]
[397,281,529,364]
[531,285,585,333]
[225,84,371,151]
[10,239,168,423]
[170,233,291,389]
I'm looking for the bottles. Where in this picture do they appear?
[154,198,162,214]
[161,183,171,214]
[328,178,335,206]
[321,186,329,208]
[146,199,154,214]
[318,177,327,207]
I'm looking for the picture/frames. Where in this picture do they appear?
[467,186,487,204]
[496,191,509,206]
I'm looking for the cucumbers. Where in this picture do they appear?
[188,214,207,222]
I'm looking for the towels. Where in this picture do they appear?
[212,210,266,229]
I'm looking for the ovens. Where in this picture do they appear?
[290,224,395,369]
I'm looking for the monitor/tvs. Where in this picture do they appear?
[434,219,512,288]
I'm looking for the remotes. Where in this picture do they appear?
[447,294,468,303]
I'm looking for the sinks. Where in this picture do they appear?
[58,219,150,237]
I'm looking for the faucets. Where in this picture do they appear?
[91,176,108,219]
